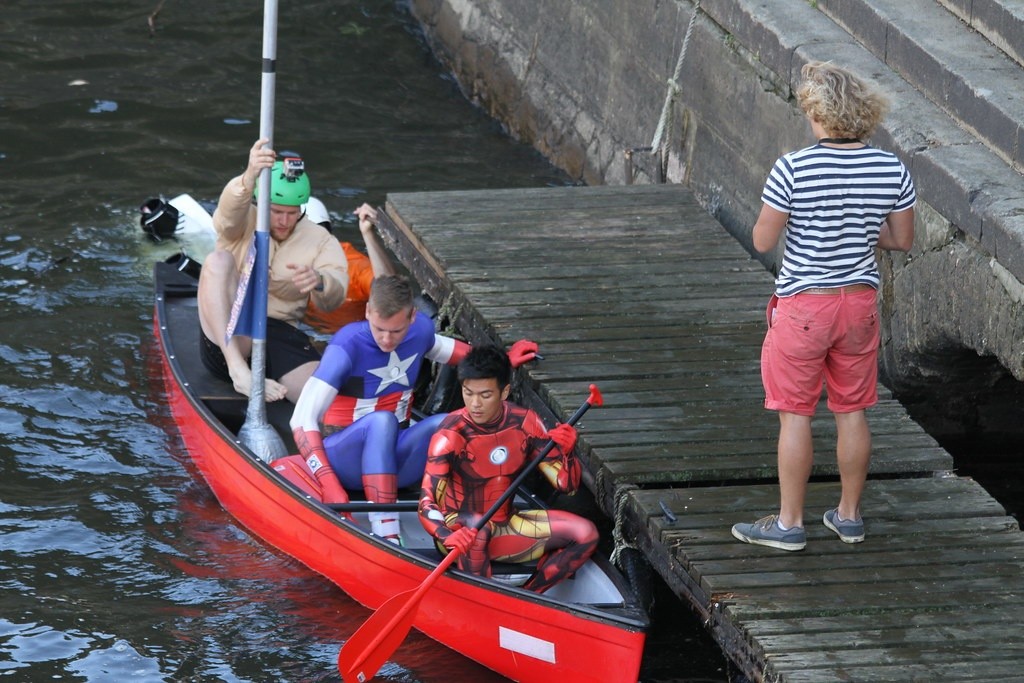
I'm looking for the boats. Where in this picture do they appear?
[153,252,651,683]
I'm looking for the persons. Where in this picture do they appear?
[290,274,539,547]
[418,344,599,595]
[197,139,350,404]
[731,61,917,551]
[297,195,396,355]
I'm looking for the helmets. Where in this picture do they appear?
[301,197,331,224]
[254,161,310,206]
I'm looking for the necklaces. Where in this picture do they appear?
[817,137,863,145]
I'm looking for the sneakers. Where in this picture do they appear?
[823,507,865,543]
[732,515,807,550]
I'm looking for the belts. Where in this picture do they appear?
[800,284,872,295]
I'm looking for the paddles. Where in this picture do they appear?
[337,383,603,683]
